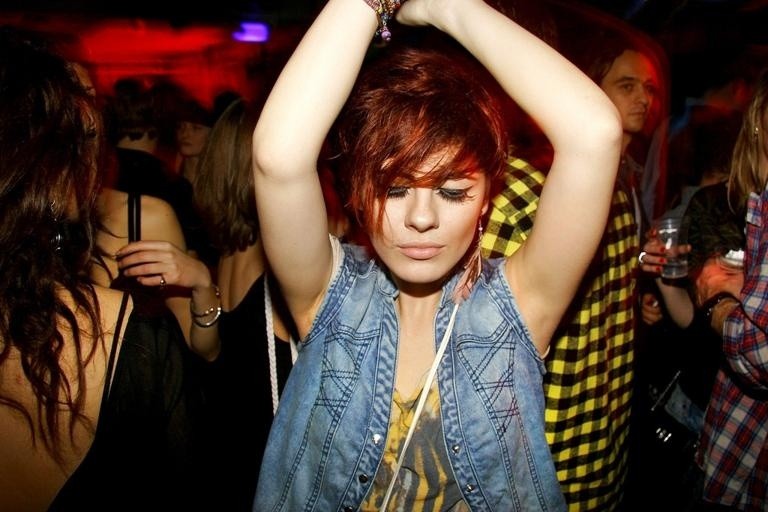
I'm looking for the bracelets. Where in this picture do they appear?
[367,0,403,41]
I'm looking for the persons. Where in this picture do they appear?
[251,0,620,511]
[480,33,768,512]
[1,44,354,512]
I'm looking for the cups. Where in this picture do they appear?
[653,217,689,278]
